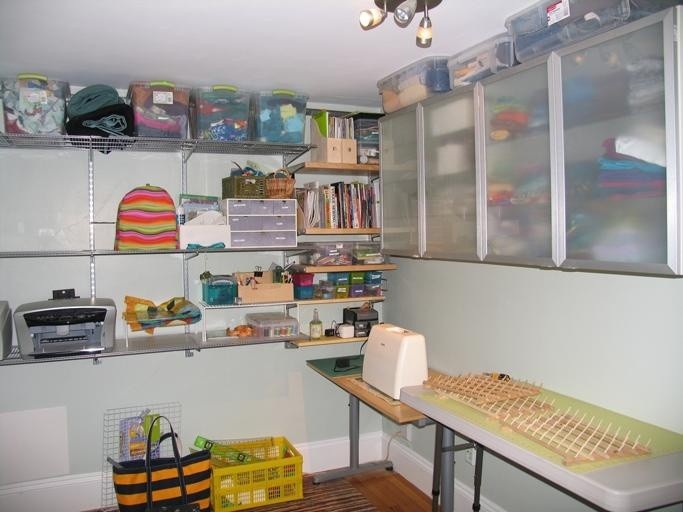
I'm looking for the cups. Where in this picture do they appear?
[325,328,333,337]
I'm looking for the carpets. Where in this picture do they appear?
[246,478,383,509]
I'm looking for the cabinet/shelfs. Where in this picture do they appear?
[422,82,482,261]
[1,139,315,371]
[285,160,396,350]
[557,12,673,274]
[378,105,424,260]
[482,46,557,267]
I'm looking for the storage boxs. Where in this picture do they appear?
[505,0,630,63]
[2,73,72,135]
[244,310,300,339]
[222,198,298,249]
[176,223,233,252]
[300,240,353,265]
[354,239,382,261]
[447,30,519,88]
[289,271,382,298]
[428,99,476,137]
[127,80,309,146]
[433,144,472,179]
[376,55,450,113]
[201,280,238,308]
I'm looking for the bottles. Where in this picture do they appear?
[308,307,324,342]
[175,202,184,225]
[331,319,337,335]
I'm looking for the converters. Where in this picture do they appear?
[325,329,334,336]
[336,358,350,367]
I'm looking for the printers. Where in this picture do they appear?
[0,300,12,361]
[13,289,117,359]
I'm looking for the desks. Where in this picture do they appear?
[395,370,682,512]
[307,351,451,510]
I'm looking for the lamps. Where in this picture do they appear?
[357,0,446,49]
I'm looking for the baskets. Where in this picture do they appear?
[221,176,264,199]
[210,436,302,512]
[265,169,294,199]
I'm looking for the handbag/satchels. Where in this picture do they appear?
[112,415,211,512]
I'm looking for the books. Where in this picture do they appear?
[297,181,376,228]
[328,116,354,139]
